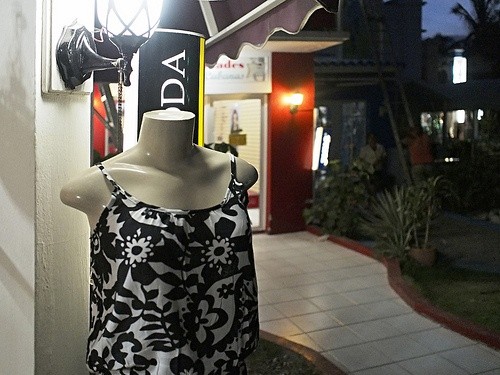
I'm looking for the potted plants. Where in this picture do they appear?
[405,175,459,268]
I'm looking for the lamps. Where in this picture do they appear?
[55,0,164,90]
[289,91,304,114]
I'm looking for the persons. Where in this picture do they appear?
[359,133,388,192]
[60,110,258,375]
